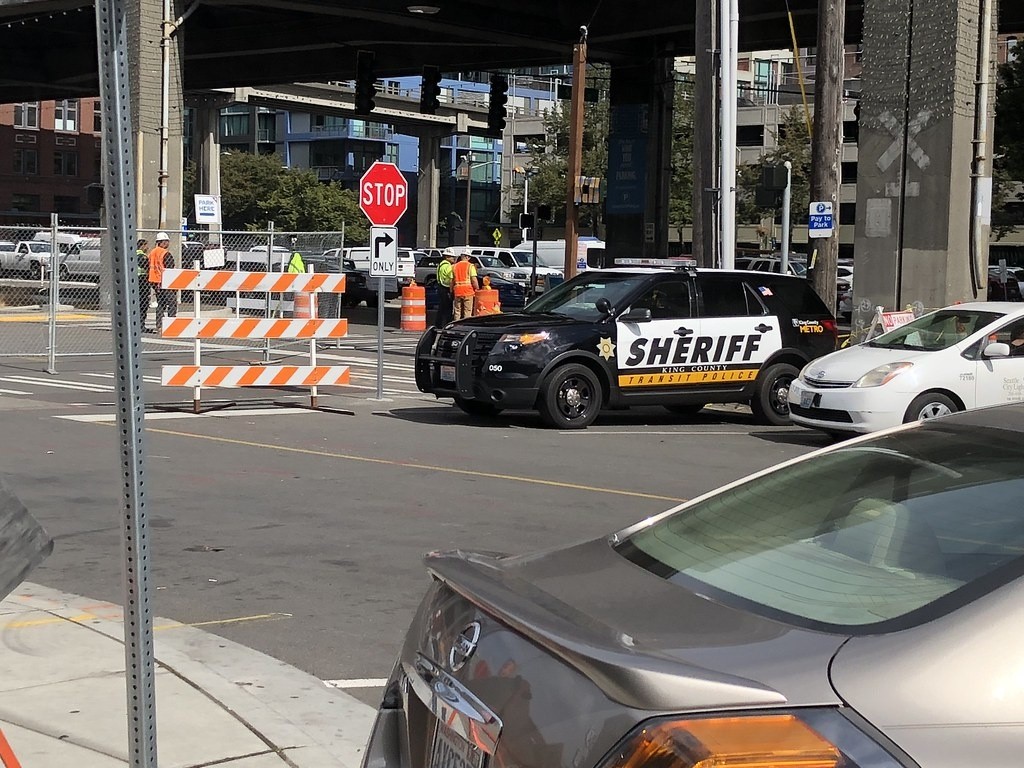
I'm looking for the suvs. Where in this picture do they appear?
[0,234,605,315]
[412,257,842,433]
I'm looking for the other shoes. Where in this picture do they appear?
[142,328,154,333]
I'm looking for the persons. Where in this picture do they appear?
[452,249,478,321]
[148,231,177,330]
[435,247,459,328]
[136,239,154,333]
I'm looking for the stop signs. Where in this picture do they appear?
[359,161,408,228]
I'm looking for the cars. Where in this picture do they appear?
[358,401,1024,767]
[789,298,1024,436]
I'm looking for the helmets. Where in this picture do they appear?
[442,248,457,256]
[155,233,170,243]
[461,249,473,257]
